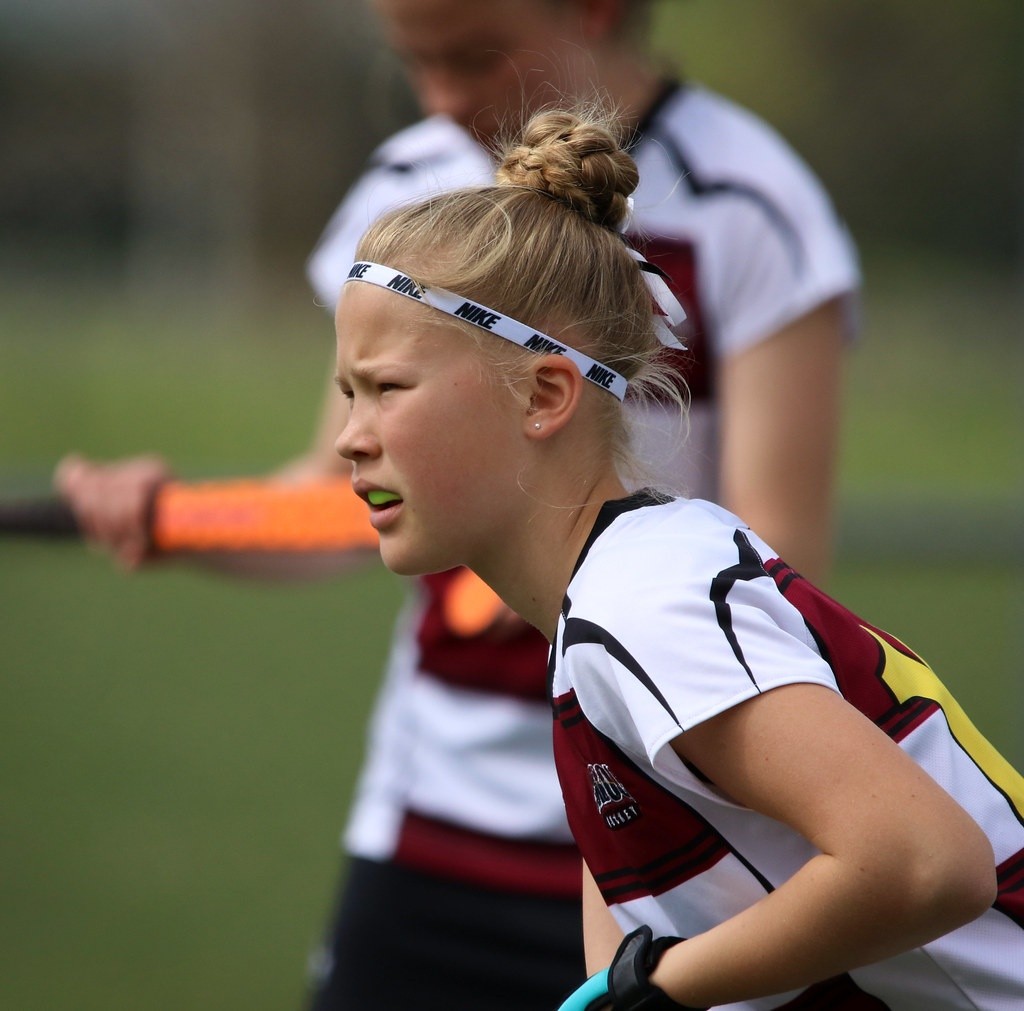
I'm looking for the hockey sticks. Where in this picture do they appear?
[3,479,515,642]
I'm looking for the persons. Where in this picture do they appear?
[335,76,1024,1011]
[51,2,864,1011]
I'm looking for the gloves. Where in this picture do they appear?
[554,925,686,1011]
[0,452,169,573]
